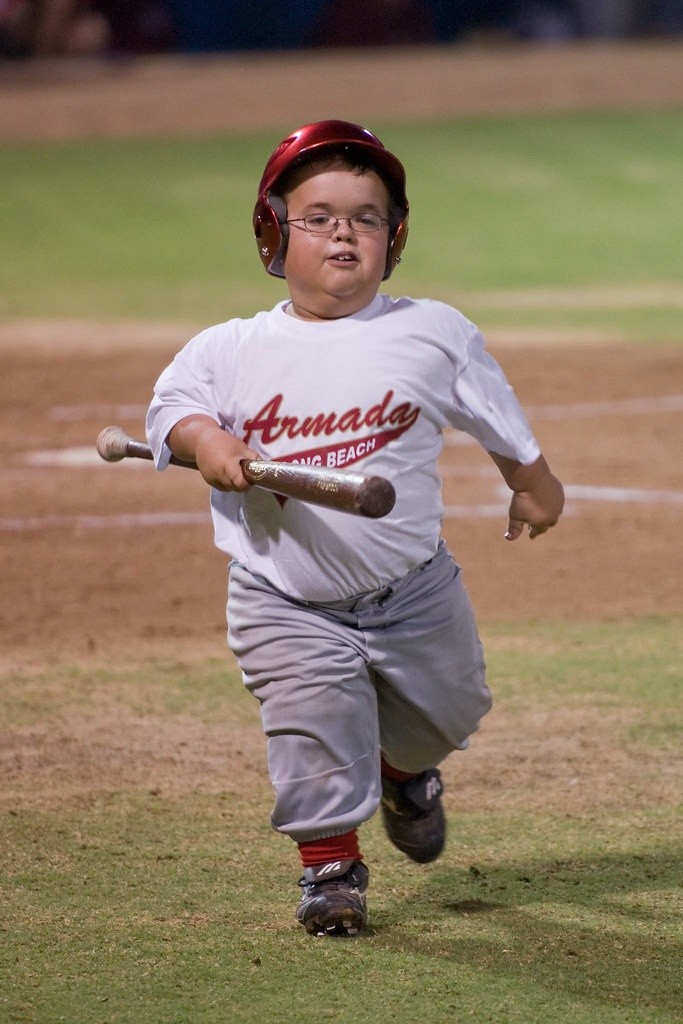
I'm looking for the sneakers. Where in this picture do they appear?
[296,859,369,938]
[380,768,446,864]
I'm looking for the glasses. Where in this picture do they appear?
[287,213,392,232]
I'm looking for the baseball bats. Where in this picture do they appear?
[92,424,399,520]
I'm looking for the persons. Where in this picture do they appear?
[145,119,564,935]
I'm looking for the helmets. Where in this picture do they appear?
[252,119,411,280]
